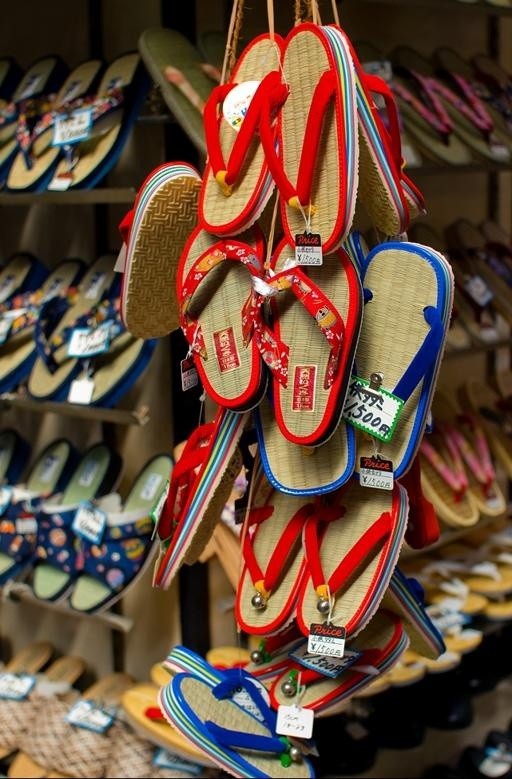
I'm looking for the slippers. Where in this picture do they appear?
[74,284,159,408]
[161,644,277,729]
[352,241,455,481]
[234,488,324,635]
[269,236,364,447]
[71,454,177,615]
[390,44,512,165]
[414,217,512,348]
[305,642,511,773]
[199,33,285,238]
[354,42,474,167]
[47,53,146,192]
[0,427,29,486]
[157,671,315,779]
[297,483,409,642]
[409,372,512,524]
[325,24,409,237]
[28,251,123,400]
[140,25,221,160]
[382,524,512,678]
[0,640,222,779]
[255,390,355,495]
[1,258,85,396]
[152,405,250,593]
[31,443,128,602]
[178,225,269,410]
[0,250,43,305]
[474,52,512,100]
[259,22,358,254]
[0,59,63,191]
[270,608,411,718]
[8,59,104,195]
[0,436,77,587]
[117,160,202,338]
